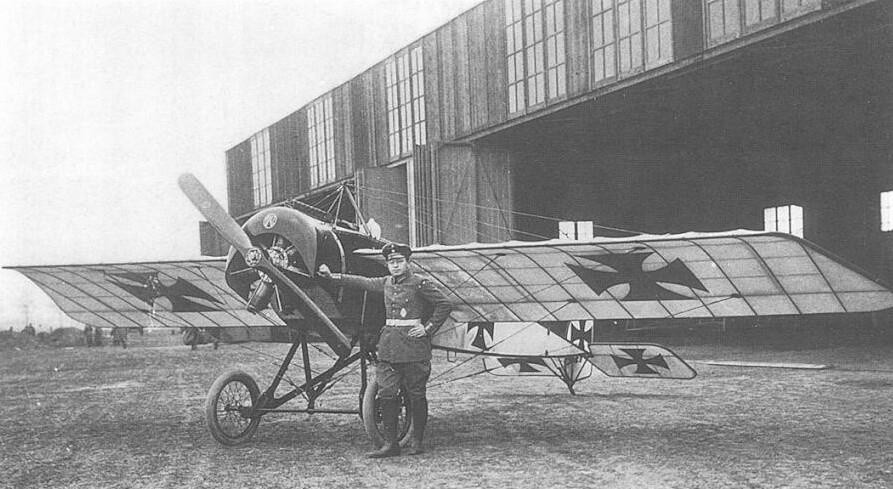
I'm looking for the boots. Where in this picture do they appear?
[408,395,427,455]
[365,399,401,459]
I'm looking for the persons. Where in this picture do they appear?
[316,242,452,459]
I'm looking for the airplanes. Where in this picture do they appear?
[0,170,893,450]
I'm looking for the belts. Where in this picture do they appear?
[384,318,421,328]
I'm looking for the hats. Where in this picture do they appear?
[382,242,412,262]
